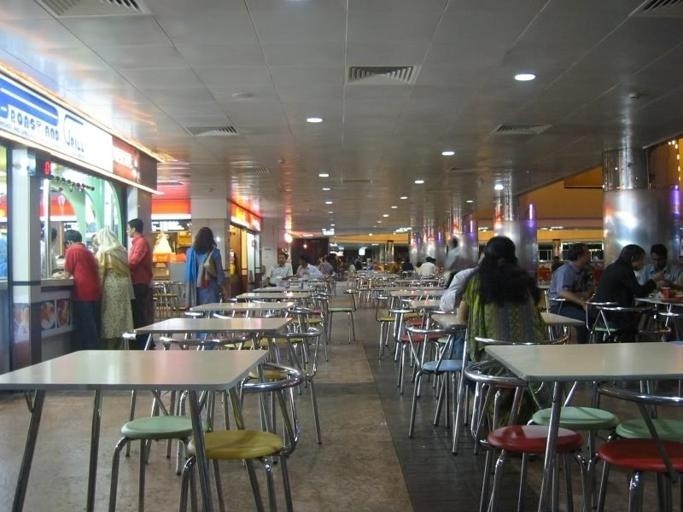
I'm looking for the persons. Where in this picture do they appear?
[39,227,58,278]
[185,226,229,339]
[95,228,136,350]
[125,218,154,350]
[388,256,440,278]
[62,230,101,351]
[440,237,548,431]
[550,242,682,342]
[266,251,369,301]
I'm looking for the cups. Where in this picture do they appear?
[660,286,670,297]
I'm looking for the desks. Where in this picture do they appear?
[0,349,270,512]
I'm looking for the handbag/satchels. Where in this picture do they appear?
[203,249,217,279]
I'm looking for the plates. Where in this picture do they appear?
[661,297,682,304]
[56,300,70,327]
[40,302,54,329]
